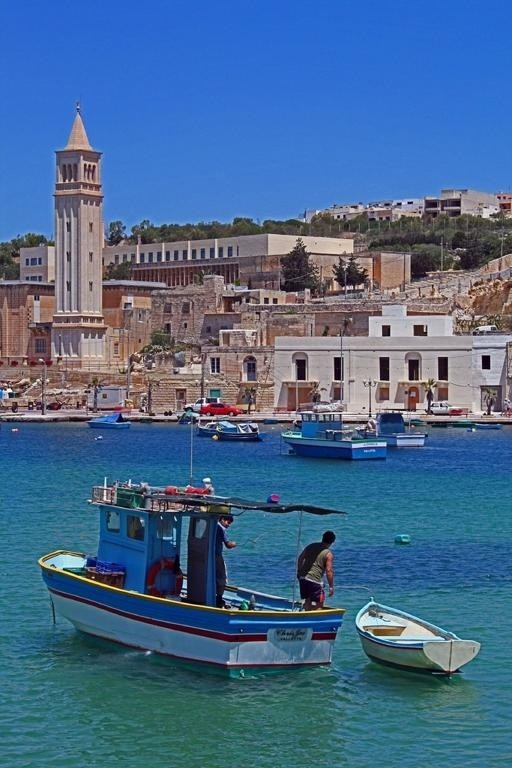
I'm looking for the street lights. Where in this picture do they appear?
[194,379,209,397]
[363,376,378,417]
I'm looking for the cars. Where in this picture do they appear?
[425,400,460,413]
[201,402,242,416]
[183,396,220,412]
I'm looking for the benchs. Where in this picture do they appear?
[273,406,291,416]
[112,406,132,416]
[449,408,468,418]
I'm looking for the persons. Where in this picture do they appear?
[214,514,237,609]
[501,398,508,417]
[296,530,336,610]
[507,400,512,417]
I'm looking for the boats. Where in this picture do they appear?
[475,422,503,429]
[197,417,260,439]
[37,475,348,666]
[355,596,481,679]
[356,408,426,447]
[281,411,388,460]
[85,413,131,429]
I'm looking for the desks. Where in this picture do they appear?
[86,567,125,589]
